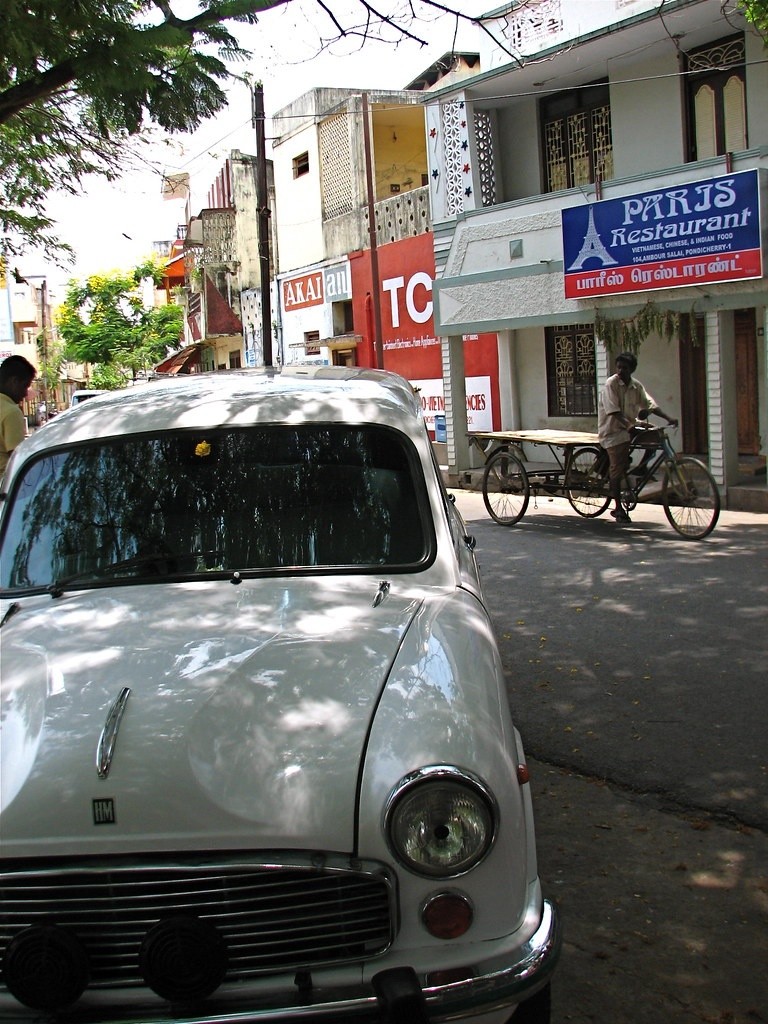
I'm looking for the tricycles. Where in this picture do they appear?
[461,416,722,539]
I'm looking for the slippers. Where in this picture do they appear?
[611,510,631,523]
[632,466,658,483]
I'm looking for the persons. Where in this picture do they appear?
[0,355,37,481]
[38,400,46,420]
[597,352,679,523]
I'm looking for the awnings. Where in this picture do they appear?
[25,389,39,402]
[153,346,200,377]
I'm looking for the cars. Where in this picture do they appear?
[1,364,562,1024]
[70,389,108,410]
